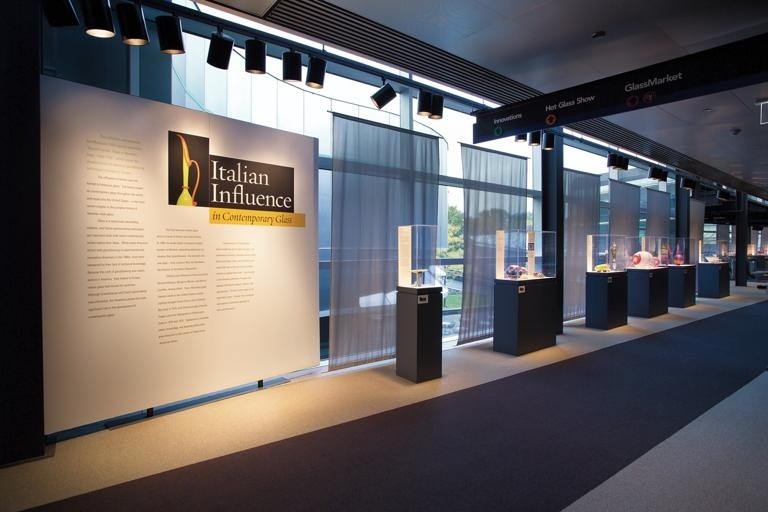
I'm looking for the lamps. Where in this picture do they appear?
[156,11,188,57]
[514,127,732,205]
[280,46,305,85]
[109,1,154,49]
[243,34,269,76]
[371,77,399,112]
[206,27,236,73]
[70,2,118,42]
[44,2,82,29]
[414,86,445,120]
[304,54,329,90]
